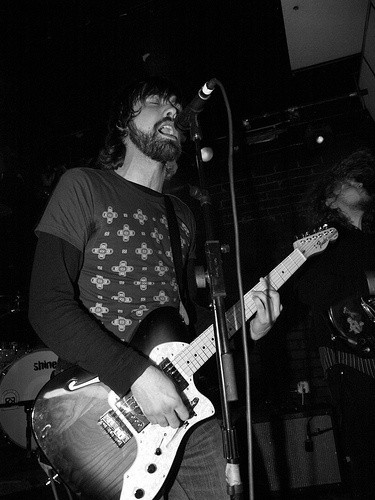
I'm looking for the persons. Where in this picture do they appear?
[301,168,375,500]
[27,76,283,500]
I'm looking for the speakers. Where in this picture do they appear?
[252,408,347,500]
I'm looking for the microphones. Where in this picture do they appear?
[305,422,314,453]
[175,79,217,133]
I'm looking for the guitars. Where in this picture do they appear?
[29,223,340,500]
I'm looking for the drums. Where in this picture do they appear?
[0,348,59,452]
